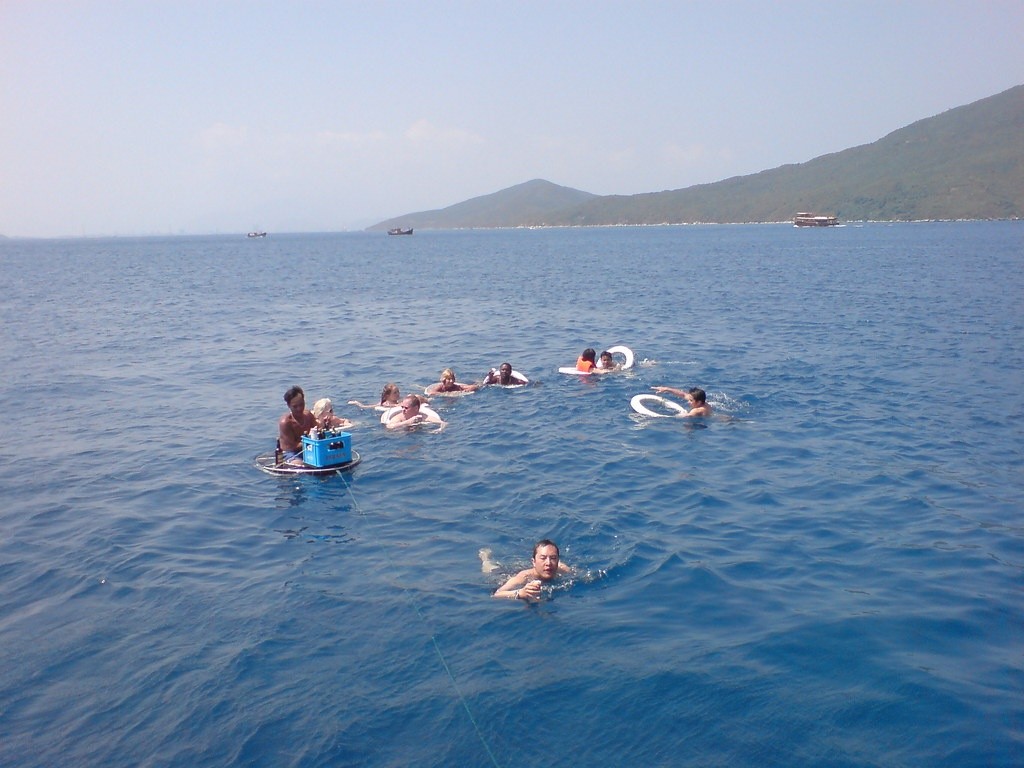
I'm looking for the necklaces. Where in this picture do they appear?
[290,412,305,428]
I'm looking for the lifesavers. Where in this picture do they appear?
[595,346,634,370]
[375,402,430,409]
[483,367,527,389]
[381,404,443,431]
[424,380,474,395]
[631,393,689,420]
[557,365,596,376]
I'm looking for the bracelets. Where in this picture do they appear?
[515,590,520,599]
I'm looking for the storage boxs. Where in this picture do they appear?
[300,431,352,467]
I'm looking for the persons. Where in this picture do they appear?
[429,369,478,395]
[598,351,621,371]
[651,386,711,417]
[485,363,525,384]
[493,540,570,600]
[380,384,399,406]
[387,396,440,427]
[279,385,322,465]
[312,398,344,429]
[575,349,602,373]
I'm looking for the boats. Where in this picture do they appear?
[791,213,840,227]
[387,225,414,235]
[247,230,268,240]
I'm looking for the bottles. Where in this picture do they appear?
[276,438,283,466]
[304,428,343,451]
